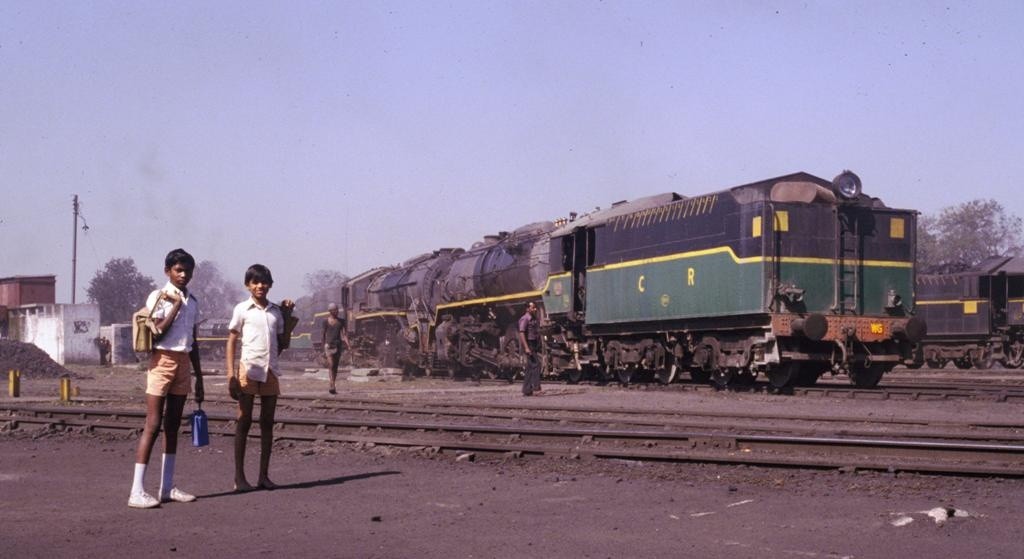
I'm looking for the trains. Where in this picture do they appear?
[297,169,929,390]
[885,253,1024,370]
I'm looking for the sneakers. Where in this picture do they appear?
[128,492,159,508]
[159,487,196,502]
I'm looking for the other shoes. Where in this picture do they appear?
[234,481,256,490]
[258,478,277,488]
[330,387,336,394]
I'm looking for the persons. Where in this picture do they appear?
[127,248,204,508]
[226,264,295,490]
[518,301,545,397]
[319,302,350,393]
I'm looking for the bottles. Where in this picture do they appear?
[191,409,209,445]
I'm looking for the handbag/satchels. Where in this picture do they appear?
[192,402,209,447]
[132,295,163,351]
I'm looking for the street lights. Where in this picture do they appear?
[69,213,90,303]
